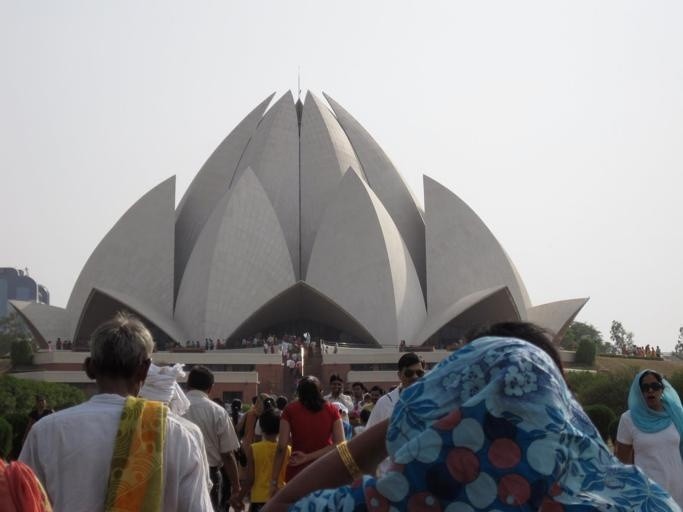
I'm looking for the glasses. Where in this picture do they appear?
[641,382,663,392]
[400,369,425,377]
